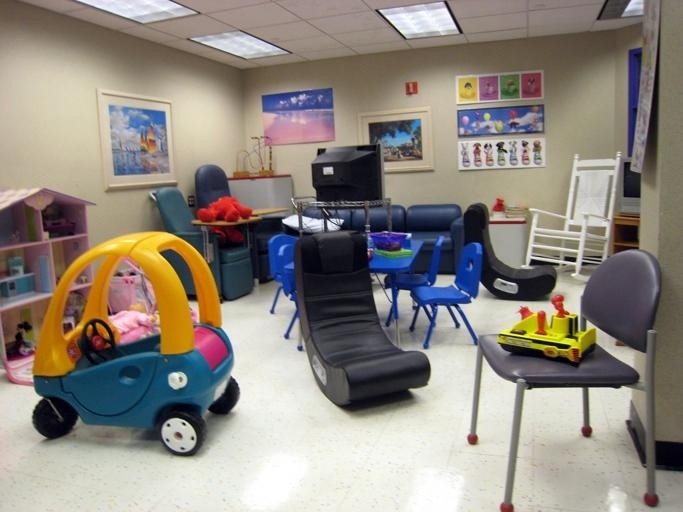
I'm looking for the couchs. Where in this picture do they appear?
[302,204,465,275]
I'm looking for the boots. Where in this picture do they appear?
[496,142,507,166]
[509,141,517,164]
[522,140,529,164]
[484,144,494,166]
[533,141,542,164]
[473,144,481,166]
[461,144,470,167]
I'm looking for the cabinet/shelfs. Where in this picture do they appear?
[0,187,97,369]
[489,217,528,268]
[290,195,392,238]
[227,174,293,217]
[611,212,640,254]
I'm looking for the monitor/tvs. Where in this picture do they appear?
[311,143,386,202]
[619,157,641,212]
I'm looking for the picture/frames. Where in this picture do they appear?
[96,88,179,193]
[357,105,435,174]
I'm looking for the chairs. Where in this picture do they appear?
[150,164,284,303]
[467,248,662,512]
[523,151,623,282]
[268,233,479,351]
[463,203,556,300]
[293,230,431,407]
